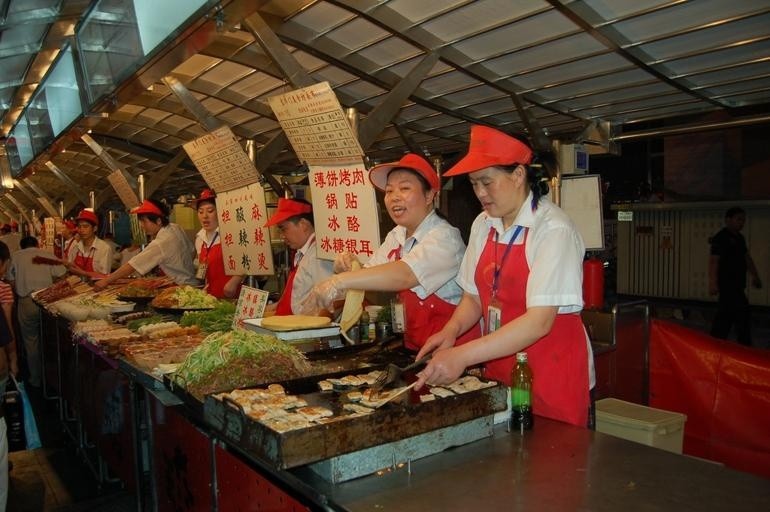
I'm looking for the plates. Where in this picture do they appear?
[149,305,214,315]
[116,294,155,303]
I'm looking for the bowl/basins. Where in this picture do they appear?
[120,334,207,370]
[55,301,136,322]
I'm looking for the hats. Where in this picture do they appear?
[367,154,440,191]
[129,200,165,216]
[262,197,312,229]
[188,191,214,209]
[443,124,533,177]
[74,211,99,225]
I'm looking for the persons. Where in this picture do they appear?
[707,205,761,346]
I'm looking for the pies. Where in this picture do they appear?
[260,315,331,332]
[339,261,366,332]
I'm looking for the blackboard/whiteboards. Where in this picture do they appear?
[542,174,605,252]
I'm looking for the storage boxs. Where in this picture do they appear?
[594,396,688,456]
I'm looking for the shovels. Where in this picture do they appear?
[376,352,432,387]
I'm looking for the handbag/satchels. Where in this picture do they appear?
[3,391,26,452]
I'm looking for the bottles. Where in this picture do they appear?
[339,302,391,346]
[583,255,604,310]
[510,352,533,431]
